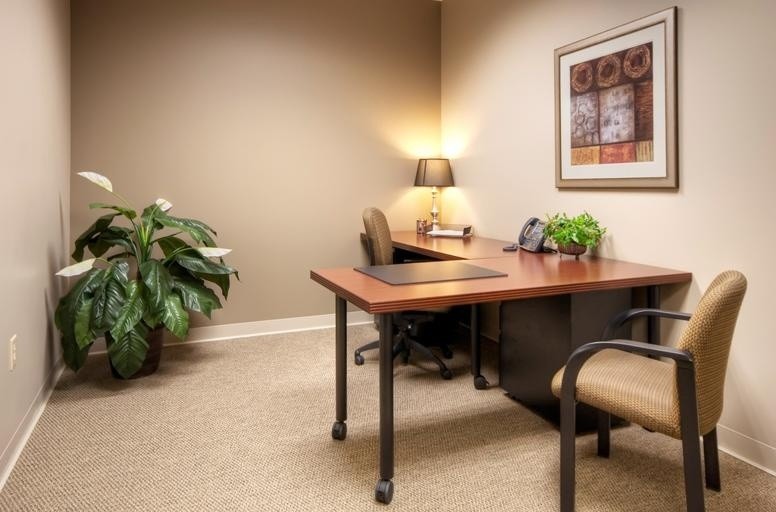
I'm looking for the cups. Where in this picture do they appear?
[417,219,428,234]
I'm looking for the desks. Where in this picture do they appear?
[310,231,691,505]
[361,230,559,259]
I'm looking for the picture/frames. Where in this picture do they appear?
[540,209,608,260]
[553,5,680,189]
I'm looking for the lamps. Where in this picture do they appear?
[414,159,455,233]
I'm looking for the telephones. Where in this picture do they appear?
[518,217,551,253]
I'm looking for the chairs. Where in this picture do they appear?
[354,207,454,380]
[550,270,747,512]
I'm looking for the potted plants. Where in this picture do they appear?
[54,171,240,380]
